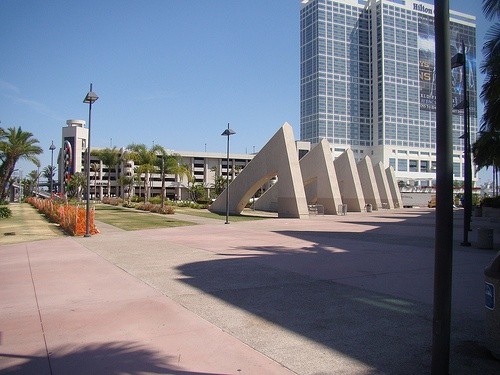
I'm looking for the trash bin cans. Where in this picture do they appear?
[476,228,497,249]
[483,253,500,360]
[366,204,372,212]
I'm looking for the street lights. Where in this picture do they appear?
[221,123,235,224]
[48,140,56,194]
[451,38,473,245]
[83,82,98,238]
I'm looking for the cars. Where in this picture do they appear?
[456,193,465,209]
[428,198,436,209]
